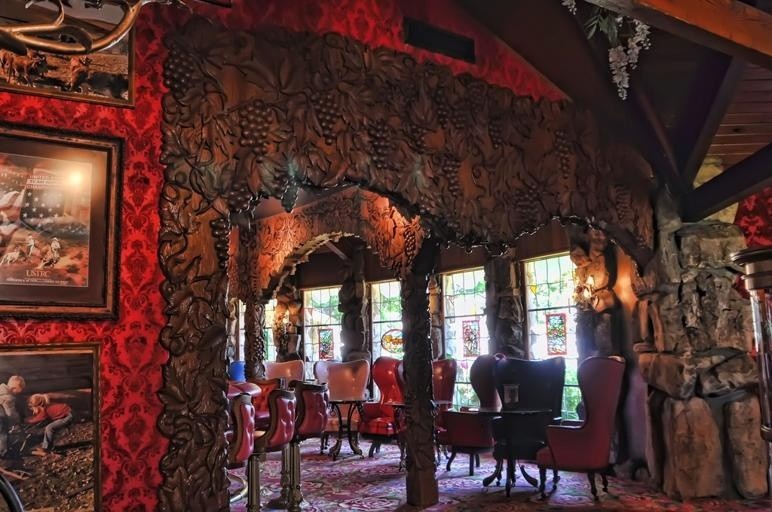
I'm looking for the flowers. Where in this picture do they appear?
[560,2,650,102]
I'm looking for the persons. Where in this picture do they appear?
[23,392,74,456]
[0,375,26,461]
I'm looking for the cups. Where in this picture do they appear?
[503,383,519,403]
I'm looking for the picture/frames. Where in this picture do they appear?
[0,0,136,109]
[0,119,126,323]
[0,342,105,512]
